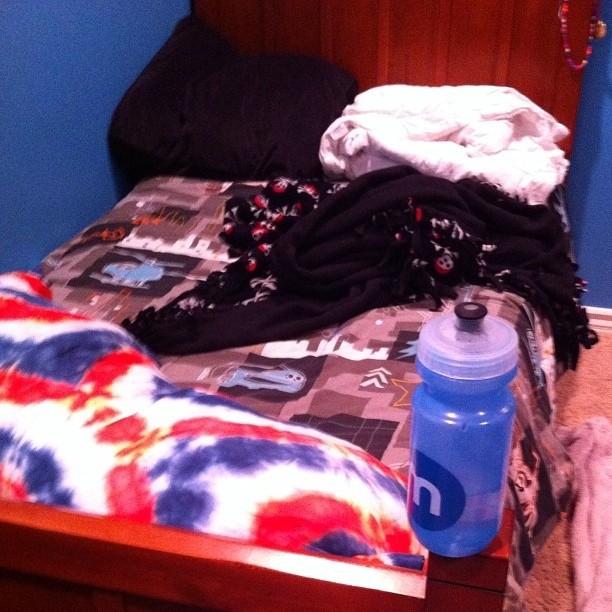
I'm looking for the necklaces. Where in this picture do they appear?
[559,0,606,72]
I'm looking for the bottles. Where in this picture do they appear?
[404,300,520,560]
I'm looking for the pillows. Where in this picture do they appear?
[0,267,424,568]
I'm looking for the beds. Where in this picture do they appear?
[0,4,600,605]
